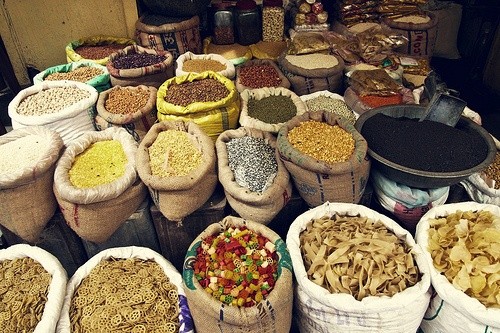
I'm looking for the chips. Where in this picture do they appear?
[428,210,500,309]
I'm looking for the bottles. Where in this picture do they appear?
[212,2,235,45]
[233,0,262,45]
[262,0,284,42]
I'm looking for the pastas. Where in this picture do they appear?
[299,213,417,301]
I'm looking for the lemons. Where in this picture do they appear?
[0,257,180,333]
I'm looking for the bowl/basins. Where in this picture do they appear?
[354,104,496,189]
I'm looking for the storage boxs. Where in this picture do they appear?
[84,197,158,257]
[146,191,230,274]
[0,211,85,280]
